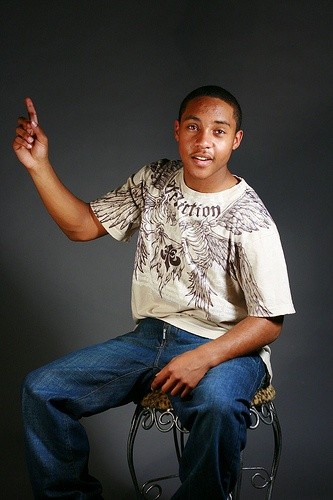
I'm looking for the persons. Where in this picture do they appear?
[0,83,298,500]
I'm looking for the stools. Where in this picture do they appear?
[126,384,283,500]
